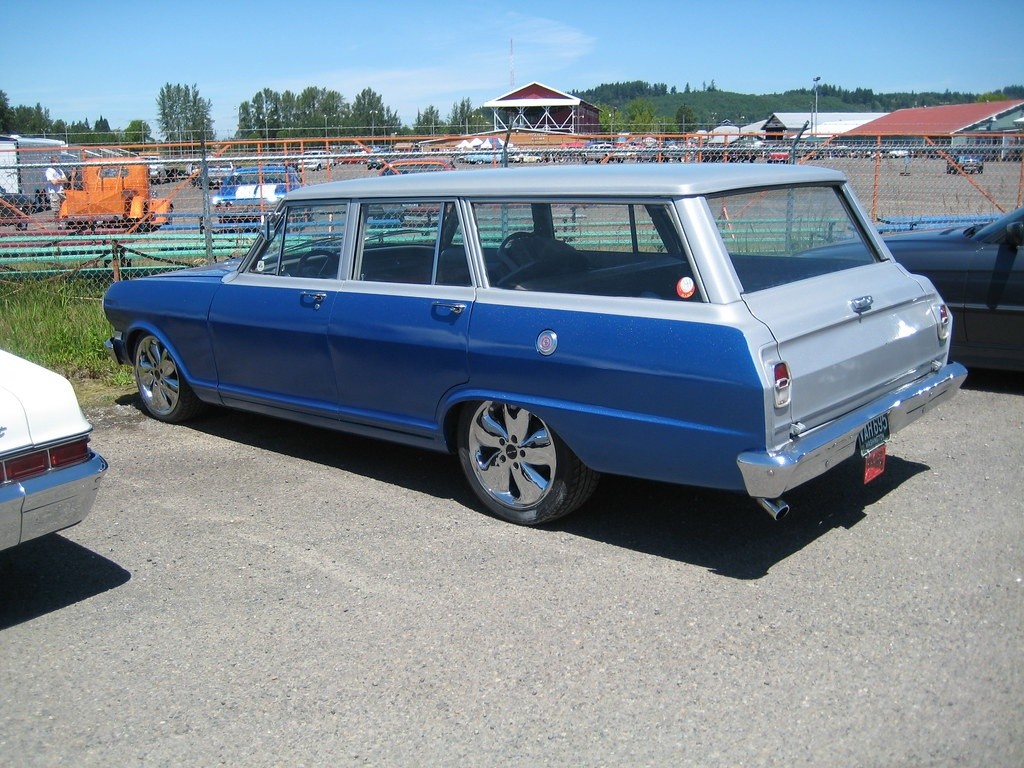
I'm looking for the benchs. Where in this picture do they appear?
[516,256,686,292]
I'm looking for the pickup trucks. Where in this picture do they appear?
[365,159,459,227]
[213,165,303,231]
[206,158,235,180]
[582,144,635,164]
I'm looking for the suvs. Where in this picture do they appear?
[953,155,983,174]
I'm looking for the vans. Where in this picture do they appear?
[298,151,333,170]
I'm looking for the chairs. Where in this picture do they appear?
[436,248,471,284]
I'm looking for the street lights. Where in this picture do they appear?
[369,110,378,148]
[814,76,820,138]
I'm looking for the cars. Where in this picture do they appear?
[102,164,969,531]
[0,187,34,229]
[367,155,388,170]
[508,152,543,164]
[0,347,108,559]
[59,159,174,234]
[336,149,368,164]
[635,145,678,163]
[792,202,1024,384]
[891,151,909,158]
[870,149,891,159]
[459,153,502,165]
